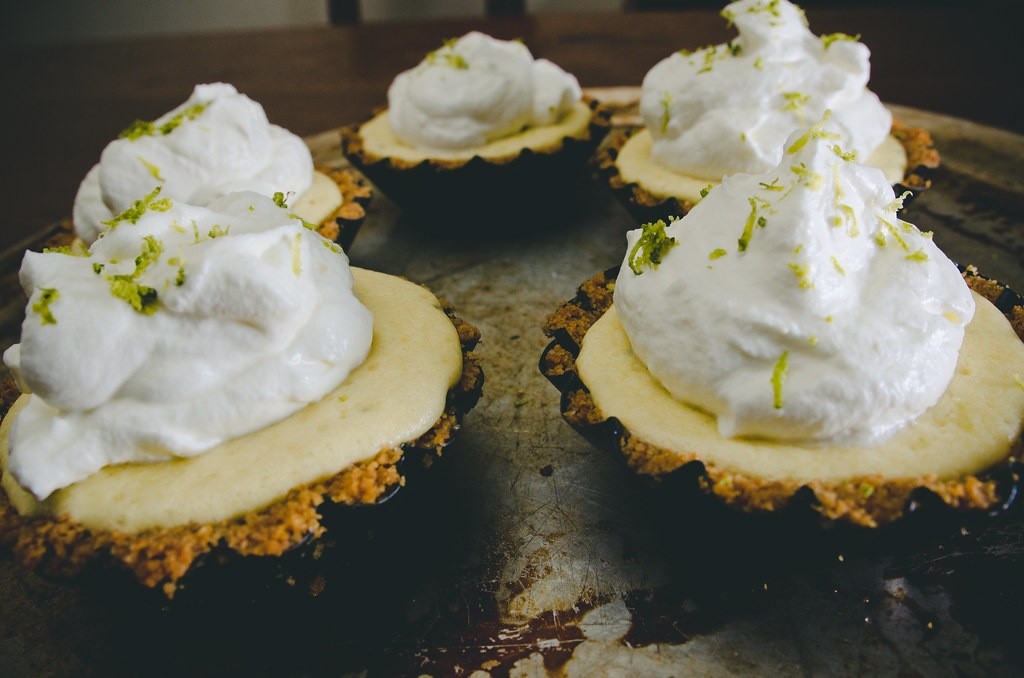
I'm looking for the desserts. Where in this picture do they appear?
[0,0,1024,633]
